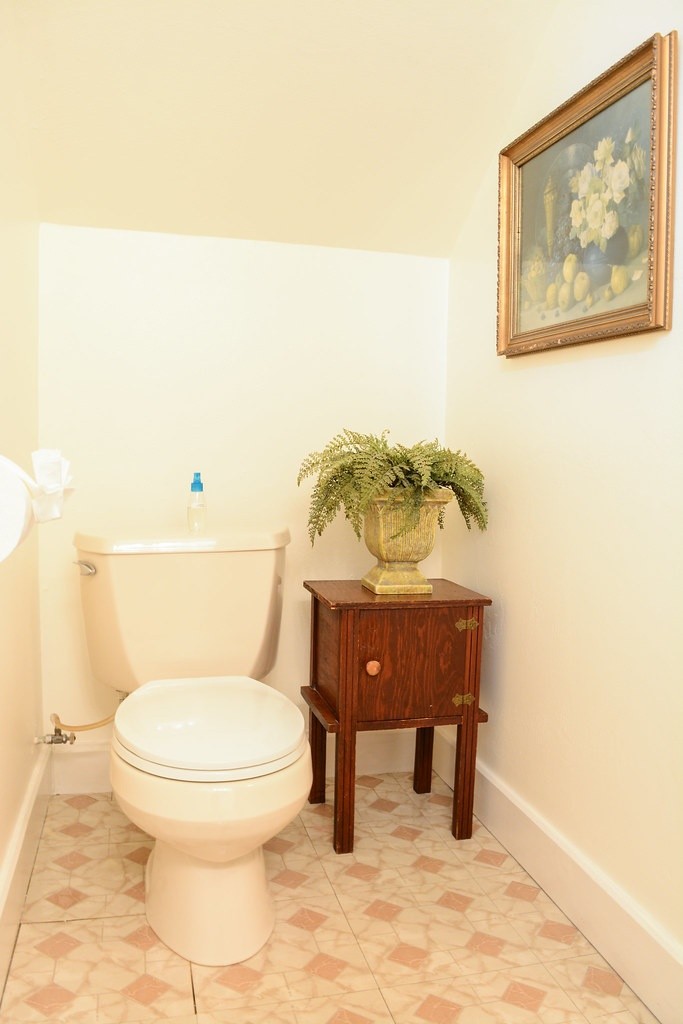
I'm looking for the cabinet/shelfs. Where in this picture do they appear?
[299,577,493,853]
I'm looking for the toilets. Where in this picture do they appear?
[75,524,315,966]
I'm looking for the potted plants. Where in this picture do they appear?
[296,427,488,594]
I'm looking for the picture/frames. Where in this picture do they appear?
[498,28,669,356]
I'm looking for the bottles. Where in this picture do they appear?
[185,473,208,545]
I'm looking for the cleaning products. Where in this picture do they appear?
[188,472,209,541]
